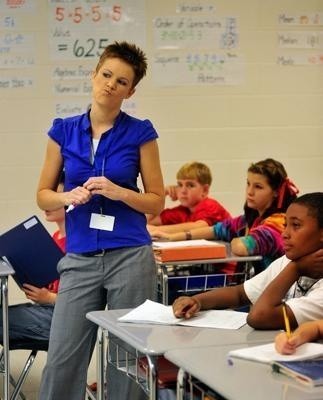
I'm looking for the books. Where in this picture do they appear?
[229,339,322,365]
[138,357,221,400]
[149,238,227,263]
[117,298,248,331]
[270,358,323,388]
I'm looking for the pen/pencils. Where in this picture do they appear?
[281,301,291,339]
[66,202,77,213]
[174,311,187,315]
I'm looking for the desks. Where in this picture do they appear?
[84,308,322,400]
[152,240,262,306]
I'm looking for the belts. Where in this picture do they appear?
[75,247,121,258]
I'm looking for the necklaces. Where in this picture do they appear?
[295,275,321,295]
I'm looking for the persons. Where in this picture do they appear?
[145,158,298,274]
[36,41,166,400]
[145,162,239,282]
[172,190,323,330]
[275,319,323,355]
[1,171,65,346]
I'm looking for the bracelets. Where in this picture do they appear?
[184,229,191,241]
